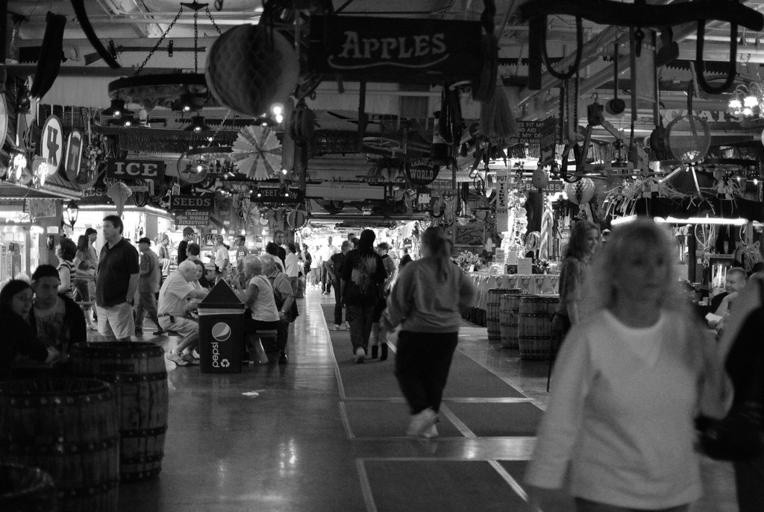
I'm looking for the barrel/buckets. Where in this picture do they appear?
[68,340,169,481]
[499,294,539,348]
[0,374,123,510]
[1,461,60,511]
[486,287,522,340]
[518,297,562,361]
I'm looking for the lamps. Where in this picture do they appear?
[98,0,223,132]
[61,199,78,230]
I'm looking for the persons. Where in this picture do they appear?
[231,230,311,367]
[214,235,229,285]
[546,217,603,394]
[158,234,171,266]
[56,215,163,342]
[157,261,211,367]
[694,261,764,512]
[400,248,411,264]
[390,227,475,438]
[311,229,395,363]
[178,227,209,288]
[705,266,747,340]
[2,264,87,369]
[523,216,735,511]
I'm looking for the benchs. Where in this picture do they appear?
[255,329,278,366]
[167,330,199,366]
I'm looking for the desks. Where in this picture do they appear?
[465,272,560,327]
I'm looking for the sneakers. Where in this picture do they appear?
[181,353,200,366]
[333,323,347,330]
[407,408,438,436]
[423,424,439,439]
[166,350,188,366]
[355,348,365,364]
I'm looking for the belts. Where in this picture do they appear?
[158,313,169,317]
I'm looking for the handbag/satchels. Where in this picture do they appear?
[274,274,298,323]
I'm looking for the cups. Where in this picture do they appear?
[488,264,503,277]
[710,263,726,290]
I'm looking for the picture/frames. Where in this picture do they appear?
[452,224,485,248]
